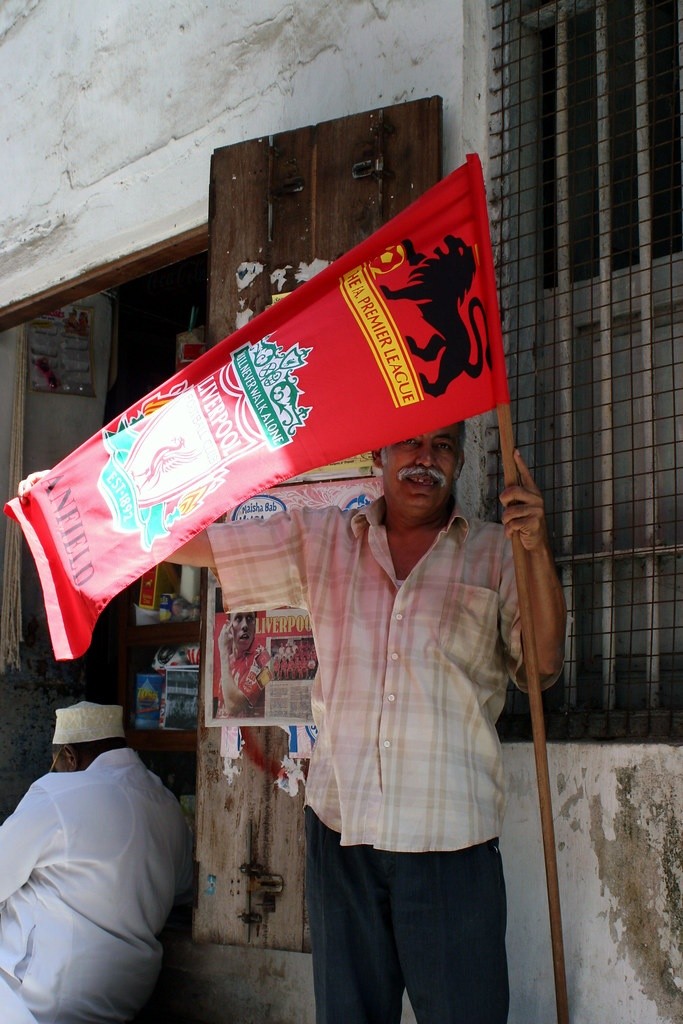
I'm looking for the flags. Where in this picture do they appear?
[5,157,506,661]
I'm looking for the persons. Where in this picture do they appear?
[217,610,270,718]
[270,639,318,678]
[16,418,568,1024]
[0,701,193,1024]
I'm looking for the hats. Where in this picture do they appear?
[52,701,125,744]
